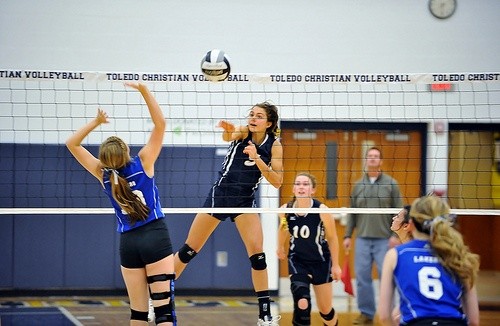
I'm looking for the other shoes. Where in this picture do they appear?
[353,314,372,324]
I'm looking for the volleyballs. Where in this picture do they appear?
[200,49,231,83]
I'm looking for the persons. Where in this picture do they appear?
[174,101,283,326]
[277,172,342,326]
[343,147,403,326]
[65,82,178,326]
[373,195,480,326]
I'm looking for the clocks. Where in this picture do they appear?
[429,0,457,19]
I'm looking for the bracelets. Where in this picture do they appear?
[249,154,261,160]
[344,236,351,239]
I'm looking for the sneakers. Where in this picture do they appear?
[257,315,281,326]
[147,298,155,322]
